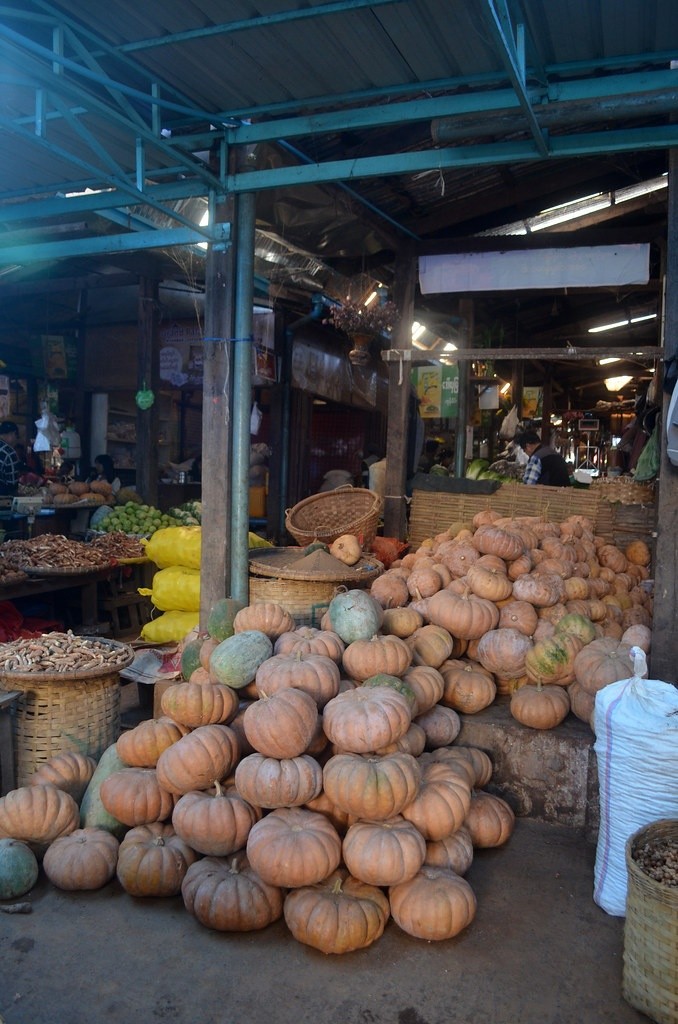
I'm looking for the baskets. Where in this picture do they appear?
[42,502,116,510]
[246,546,384,629]
[0,634,133,789]
[0,551,110,576]
[283,483,382,551]
[114,555,150,565]
[0,571,27,587]
[619,818,678,1024]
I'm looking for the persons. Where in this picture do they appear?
[57,462,75,484]
[86,453,121,489]
[420,438,441,472]
[519,431,571,488]
[437,450,455,470]
[0,422,21,493]
[187,452,202,482]
[366,447,391,538]
[316,468,356,494]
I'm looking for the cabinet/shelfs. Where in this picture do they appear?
[90,389,174,471]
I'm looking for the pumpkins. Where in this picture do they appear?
[31,474,117,504]
[0,500,654,956]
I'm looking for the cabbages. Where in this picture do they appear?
[467,457,526,485]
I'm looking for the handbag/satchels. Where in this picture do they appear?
[615,405,662,479]
